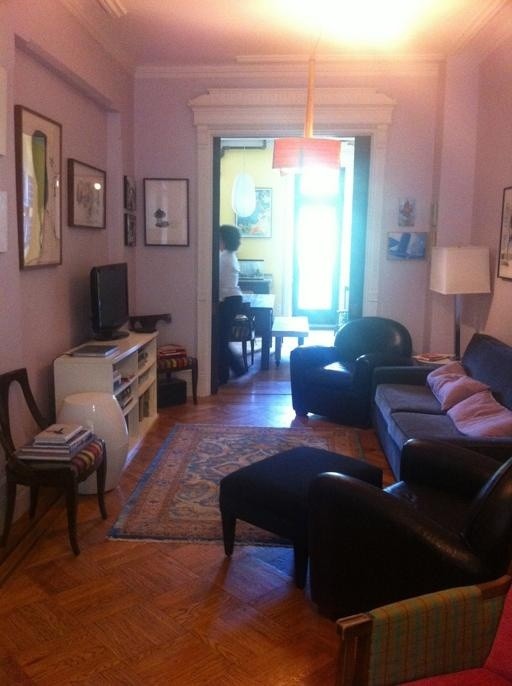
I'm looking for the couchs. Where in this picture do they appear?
[371,333,511,482]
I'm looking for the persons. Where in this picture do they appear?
[219,224,247,385]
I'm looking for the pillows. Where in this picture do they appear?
[446,391,511,440]
[426,360,491,412]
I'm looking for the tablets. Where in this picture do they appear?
[72,345,118,357]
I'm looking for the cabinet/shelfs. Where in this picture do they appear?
[52,331,160,471]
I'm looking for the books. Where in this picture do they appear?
[17,344,150,462]
[416,353,447,362]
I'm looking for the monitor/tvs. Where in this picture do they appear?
[91,262,130,341]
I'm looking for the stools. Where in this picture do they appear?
[220,443,384,589]
[271,315,310,368]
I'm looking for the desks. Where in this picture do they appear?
[243,293,275,370]
[238,274,273,294]
[412,352,445,366]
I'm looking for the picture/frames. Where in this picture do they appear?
[13,104,62,271]
[496,187,512,283]
[429,202,437,227]
[125,212,137,247]
[429,230,436,245]
[234,188,273,239]
[387,231,427,259]
[68,157,106,229]
[143,178,190,247]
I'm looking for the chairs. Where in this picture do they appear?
[289,317,418,429]
[228,302,257,371]
[333,575,511,685]
[309,436,511,622]
[129,312,200,405]
[0,368,109,556]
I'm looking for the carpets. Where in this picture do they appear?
[105,423,398,548]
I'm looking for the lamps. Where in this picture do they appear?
[429,242,491,360]
[271,55,341,174]
